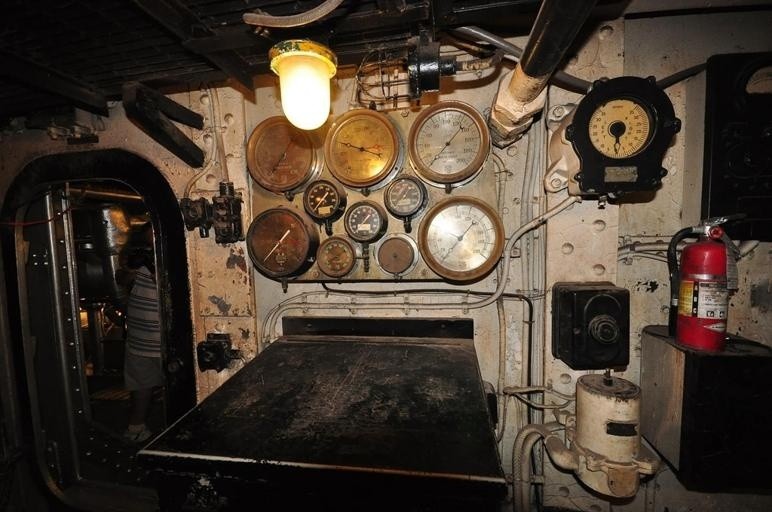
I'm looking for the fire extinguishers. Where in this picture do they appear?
[666,217,742,352]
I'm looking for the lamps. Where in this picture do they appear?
[273,41,338,130]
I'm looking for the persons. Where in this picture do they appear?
[119,224,166,441]
